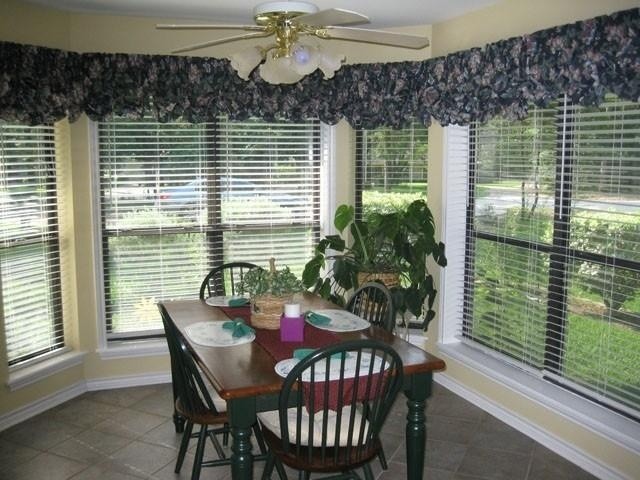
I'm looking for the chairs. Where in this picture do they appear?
[347,279,399,468]
[253,336,408,480]
[154,301,293,480]
[199,263,270,303]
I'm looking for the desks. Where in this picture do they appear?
[157,287,448,480]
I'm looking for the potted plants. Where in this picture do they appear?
[295,192,447,333]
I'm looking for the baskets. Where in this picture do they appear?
[357,271,400,313]
[247,270,292,331]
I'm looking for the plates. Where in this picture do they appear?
[303,309,371,333]
[273,351,391,383]
[204,294,252,309]
[180,319,258,348]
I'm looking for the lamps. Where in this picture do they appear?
[222,38,345,85]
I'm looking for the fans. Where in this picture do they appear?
[153,2,432,56]
